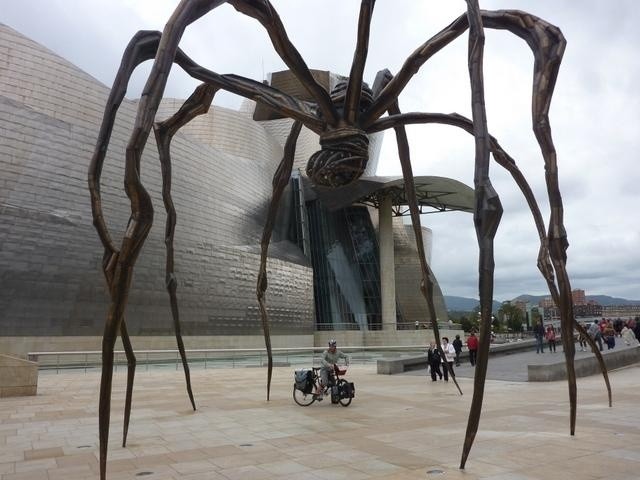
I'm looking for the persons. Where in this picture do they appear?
[448,319,453,330]
[533,320,557,353]
[312,340,351,401]
[414,319,433,329]
[427,332,479,381]
[576,316,640,352]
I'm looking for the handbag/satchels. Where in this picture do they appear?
[447,352,456,358]
[295,370,312,393]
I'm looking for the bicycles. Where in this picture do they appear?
[293,363,355,407]
